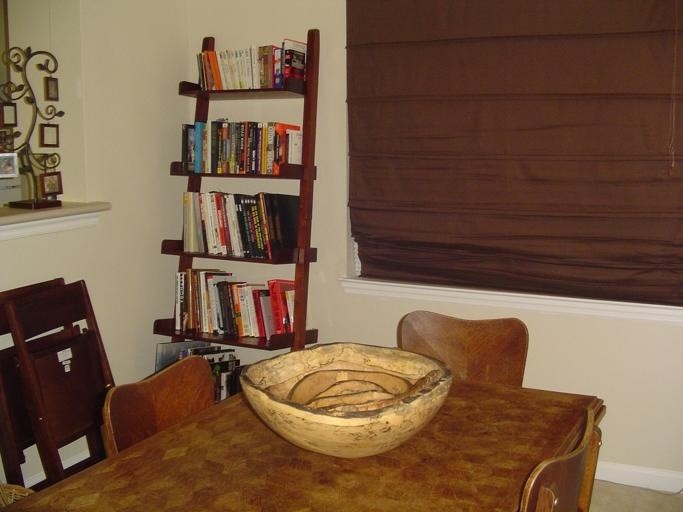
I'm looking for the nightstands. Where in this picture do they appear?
[152,27,320,353]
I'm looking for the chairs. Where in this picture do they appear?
[103,355,215,458]
[6,280,116,484]
[520,408,605,512]
[0,276,103,492]
[396,310,531,385]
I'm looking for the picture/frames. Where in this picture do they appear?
[44,77,59,102]
[39,124,59,148]
[0,153,19,179]
[40,171,63,196]
[0,103,17,127]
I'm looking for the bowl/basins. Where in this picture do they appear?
[237,340,454,459]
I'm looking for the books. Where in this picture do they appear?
[194,39,306,91]
[174,267,294,337]
[181,190,299,261]
[153,340,242,399]
[178,116,302,177]
